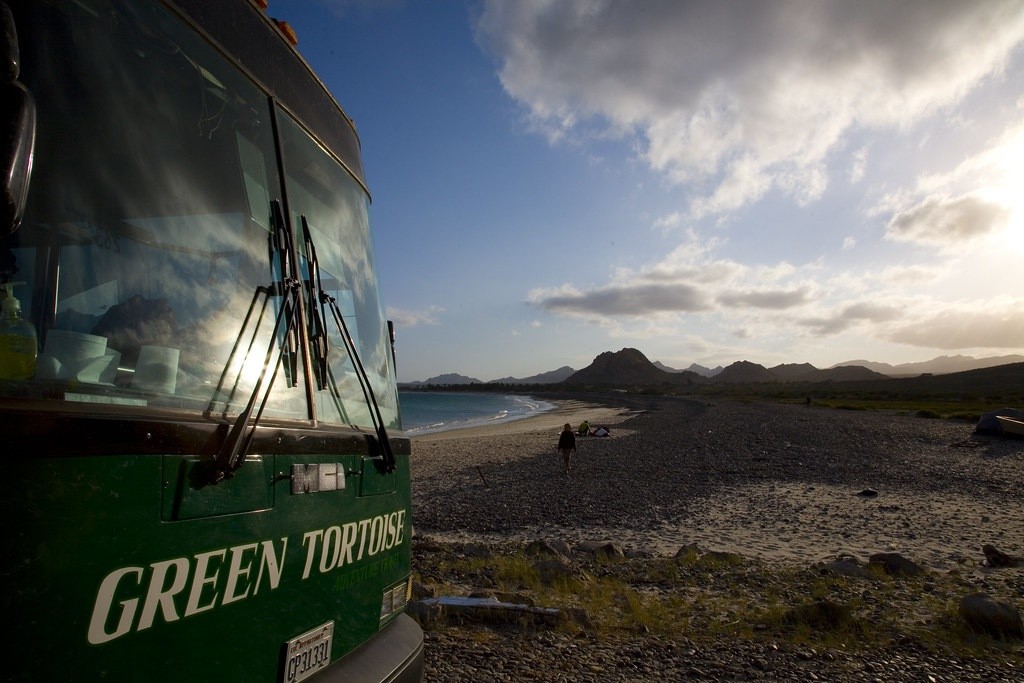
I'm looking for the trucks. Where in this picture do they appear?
[0,1,429,683]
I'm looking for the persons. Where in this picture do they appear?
[578,421,591,437]
[558,423,576,473]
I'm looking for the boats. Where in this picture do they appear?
[995,414,1024,435]
